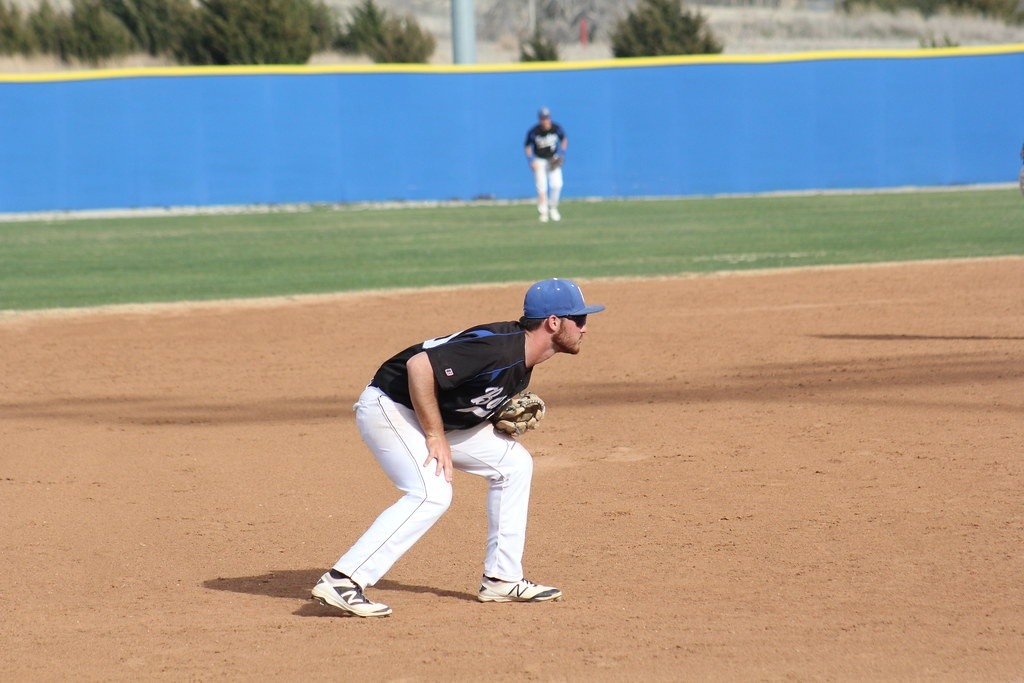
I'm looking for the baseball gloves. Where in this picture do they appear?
[494,391,546,437]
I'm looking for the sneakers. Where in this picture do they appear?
[477,572,562,603]
[310,571,392,617]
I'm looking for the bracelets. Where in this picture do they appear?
[528,157,533,162]
[557,149,565,157]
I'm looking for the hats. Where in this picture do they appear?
[523,278,606,319]
[539,108,549,117]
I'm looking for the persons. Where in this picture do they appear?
[309,279,603,618]
[525,107,568,223]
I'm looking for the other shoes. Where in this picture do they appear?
[540,212,548,223]
[549,205,560,223]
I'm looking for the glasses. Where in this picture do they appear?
[567,315,587,328]
